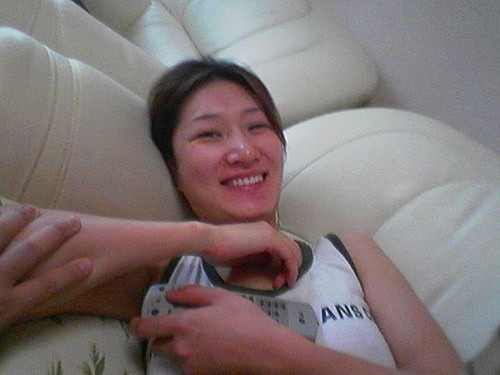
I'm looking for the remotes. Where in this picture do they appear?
[140,284,319,343]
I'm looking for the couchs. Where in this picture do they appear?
[0,0,500,375]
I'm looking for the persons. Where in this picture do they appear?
[0,205,95,335]
[0,53,471,375]
